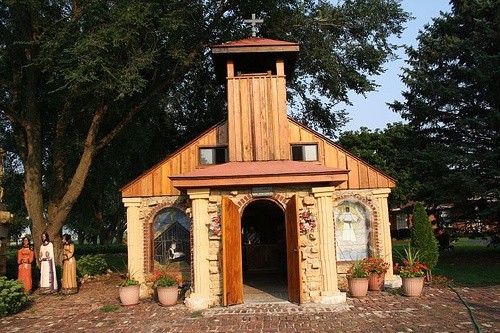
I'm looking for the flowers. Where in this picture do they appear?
[205,217,221,238]
[148,267,183,288]
[346,257,390,280]
[299,210,316,233]
[393,243,428,278]
[112,261,143,287]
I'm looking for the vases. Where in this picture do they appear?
[402,276,426,297]
[368,272,386,290]
[348,278,369,297]
[118,284,140,305]
[157,286,178,306]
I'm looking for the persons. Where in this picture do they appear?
[61,234,77,290]
[168,243,176,263]
[39,233,57,290]
[17,236,34,292]
